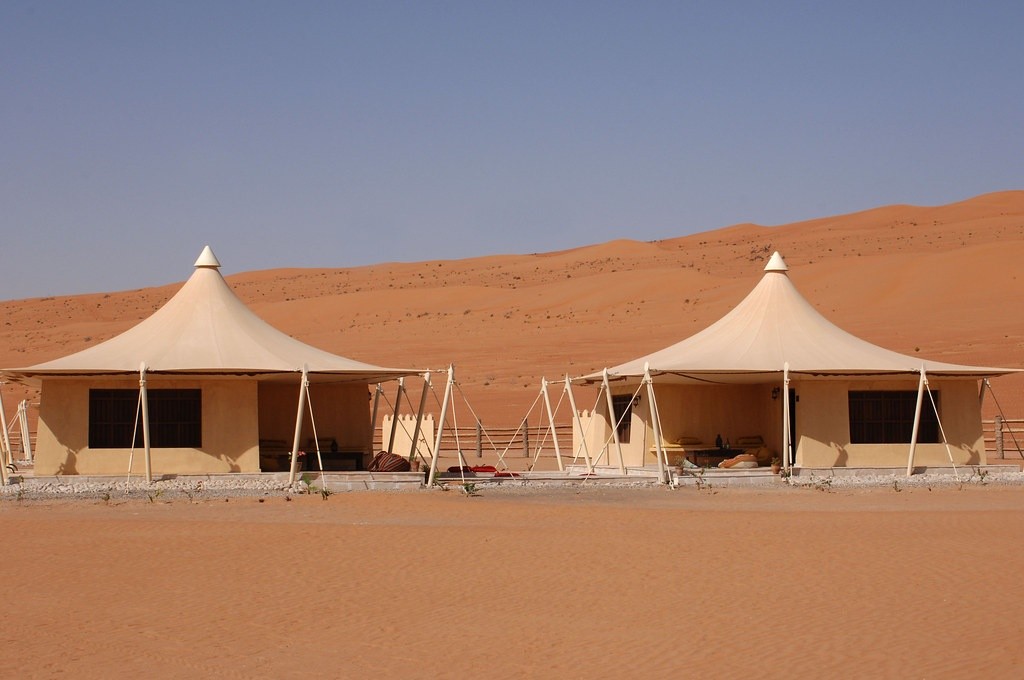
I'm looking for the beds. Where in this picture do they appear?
[260,437,372,470]
[649,435,767,464]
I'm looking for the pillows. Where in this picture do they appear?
[673,437,703,445]
[735,436,764,444]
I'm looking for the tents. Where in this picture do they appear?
[534,250,1023,482]
[0,246,453,492]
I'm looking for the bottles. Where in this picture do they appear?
[724,437,730,449]
[716,434,722,447]
